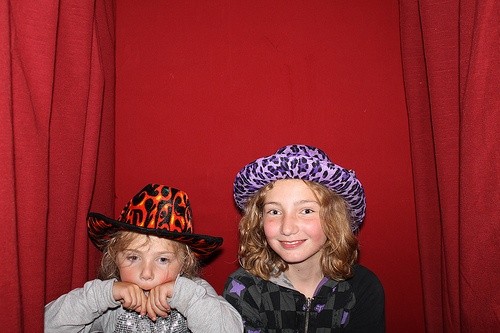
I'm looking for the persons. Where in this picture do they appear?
[221,141,389,333]
[41,185,245,333]
